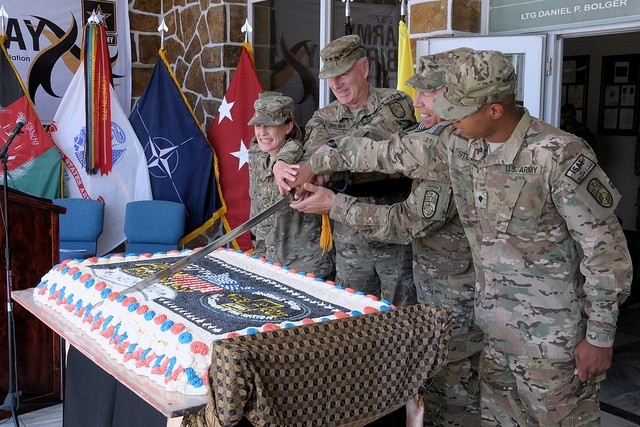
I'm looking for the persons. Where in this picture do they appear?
[289,48,480,426]
[249,96,334,278]
[284,51,633,426]
[297,34,416,304]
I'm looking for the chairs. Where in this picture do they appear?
[168,303,452,427]
[124,200,186,255]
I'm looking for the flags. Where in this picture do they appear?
[47,10,153,257]
[129,18,227,245]
[0,5,63,199]
[207,18,264,249]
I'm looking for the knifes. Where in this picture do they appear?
[88,185,300,310]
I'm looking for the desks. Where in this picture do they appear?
[10,288,210,426]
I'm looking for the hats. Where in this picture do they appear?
[247,96,295,127]
[317,34,367,79]
[431,50,518,121]
[258,91,283,98]
[405,47,476,92]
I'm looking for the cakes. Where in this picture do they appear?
[33,246,398,395]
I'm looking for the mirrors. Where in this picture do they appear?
[51,199,104,262]
